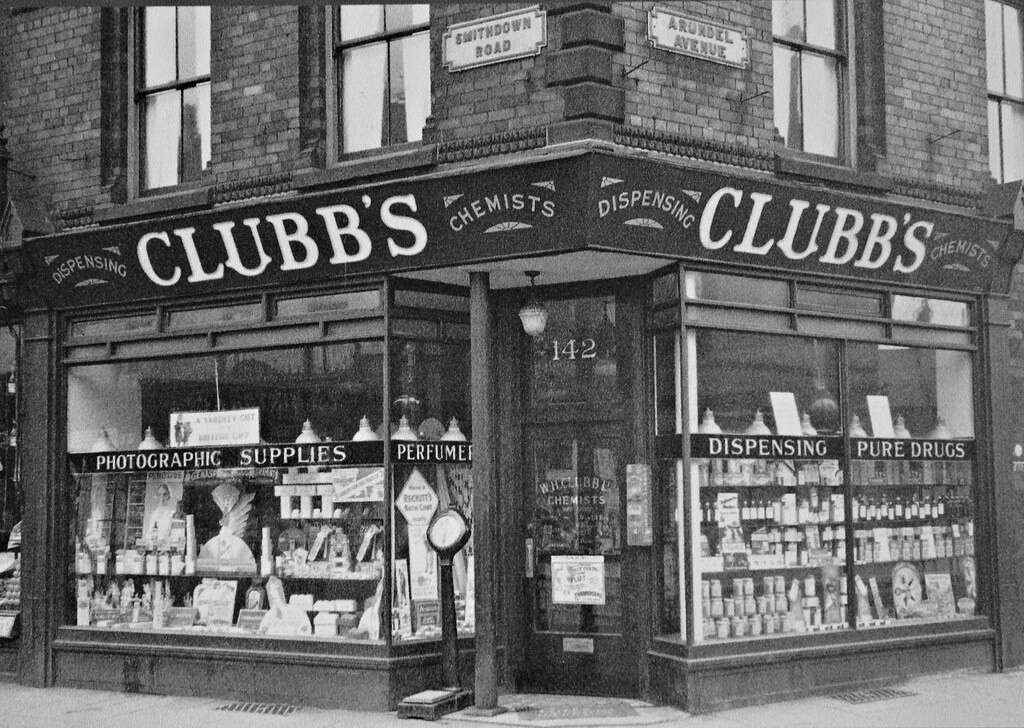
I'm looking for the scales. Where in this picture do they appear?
[395,506,472,721]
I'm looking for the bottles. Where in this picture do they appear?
[858,494,975,521]
[738,500,777,525]
[699,499,718,528]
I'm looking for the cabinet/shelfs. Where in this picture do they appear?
[699,458,976,642]
[68,472,386,642]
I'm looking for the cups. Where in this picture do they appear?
[144,553,183,577]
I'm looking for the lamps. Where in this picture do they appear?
[519,271,548,338]
